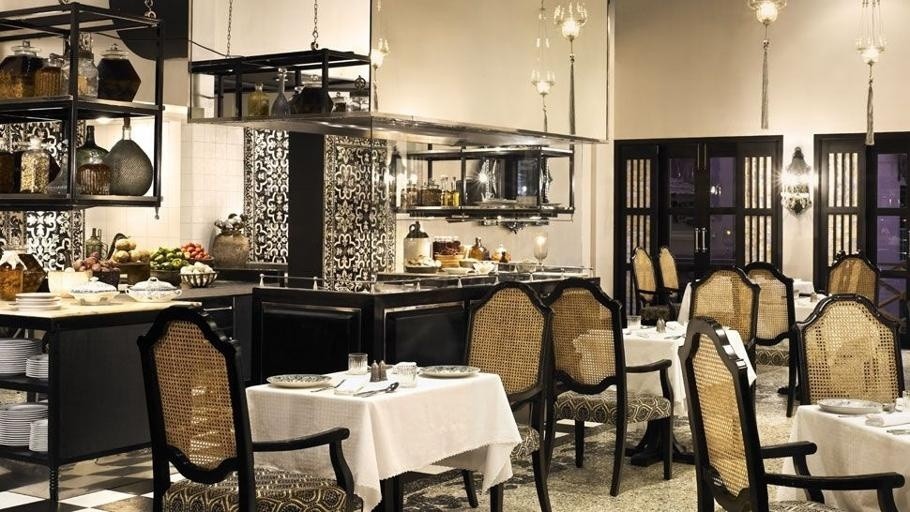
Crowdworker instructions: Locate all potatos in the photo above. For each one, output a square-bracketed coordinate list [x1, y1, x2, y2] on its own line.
[112, 238, 151, 263]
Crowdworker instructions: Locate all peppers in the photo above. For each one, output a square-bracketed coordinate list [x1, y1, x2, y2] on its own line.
[149, 246, 188, 271]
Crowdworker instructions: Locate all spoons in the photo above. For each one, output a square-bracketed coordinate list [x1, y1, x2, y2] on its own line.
[353, 382, 398, 398]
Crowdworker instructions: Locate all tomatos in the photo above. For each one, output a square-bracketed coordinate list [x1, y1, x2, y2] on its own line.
[181, 242, 211, 260]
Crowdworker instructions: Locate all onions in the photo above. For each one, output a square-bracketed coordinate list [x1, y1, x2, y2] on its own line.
[72, 251, 120, 273]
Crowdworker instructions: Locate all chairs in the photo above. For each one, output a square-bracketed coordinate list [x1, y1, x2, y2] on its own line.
[540, 276, 676, 498]
[135, 303, 365, 512]
[651, 243, 685, 321]
[689, 264, 762, 419]
[630, 245, 683, 328]
[678, 315, 910, 512]
[743, 260, 798, 418]
[790, 292, 904, 406]
[461, 280, 554, 512]
[825, 253, 881, 311]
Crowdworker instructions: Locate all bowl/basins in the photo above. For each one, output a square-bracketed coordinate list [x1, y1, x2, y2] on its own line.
[473, 263, 494, 274]
[458, 260, 476, 268]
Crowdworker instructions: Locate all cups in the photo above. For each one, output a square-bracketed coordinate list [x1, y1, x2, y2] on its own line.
[627, 316, 642, 330]
[397, 362, 417, 388]
[348, 353, 368, 374]
[901, 390, 909, 415]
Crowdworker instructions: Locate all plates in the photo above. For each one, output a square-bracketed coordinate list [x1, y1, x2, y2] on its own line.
[266, 374, 332, 388]
[817, 398, 883, 414]
[29, 418, 48, 452]
[0, 403, 48, 446]
[0, 338, 42, 378]
[442, 267, 472, 274]
[8, 293, 62, 311]
[435, 254, 465, 268]
[25, 354, 49, 383]
[419, 365, 481, 378]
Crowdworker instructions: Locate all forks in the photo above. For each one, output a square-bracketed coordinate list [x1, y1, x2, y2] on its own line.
[310, 379, 346, 392]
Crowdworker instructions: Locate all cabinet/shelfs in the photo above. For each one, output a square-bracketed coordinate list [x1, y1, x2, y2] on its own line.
[0, 0, 167, 220]
[187, 1, 611, 145]
[1, 299, 201, 507]
[395, 142, 576, 234]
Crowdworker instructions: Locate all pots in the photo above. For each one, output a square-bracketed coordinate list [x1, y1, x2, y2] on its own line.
[68, 277, 120, 305]
[124, 277, 182, 303]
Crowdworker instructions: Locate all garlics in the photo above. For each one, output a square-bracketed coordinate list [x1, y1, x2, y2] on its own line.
[180, 261, 217, 286]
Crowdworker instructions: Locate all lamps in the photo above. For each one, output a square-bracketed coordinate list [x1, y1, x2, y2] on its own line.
[371, 1, 389, 112]
[530, 1, 557, 133]
[533, 229, 549, 266]
[778, 146, 815, 218]
[856, 0, 891, 147]
[551, 1, 589, 135]
[746, 1, 789, 132]
